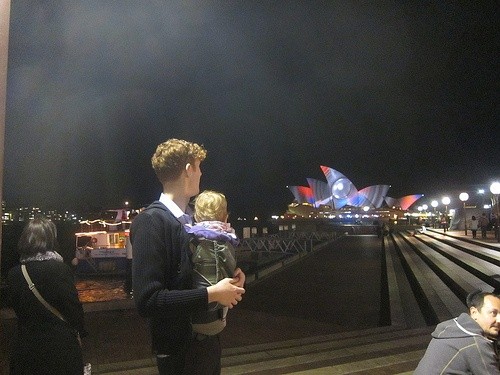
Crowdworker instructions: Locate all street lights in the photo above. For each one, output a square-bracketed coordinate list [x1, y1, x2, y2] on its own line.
[459, 192, 469, 236]
[490, 181, 500, 243]
[441, 196, 451, 231]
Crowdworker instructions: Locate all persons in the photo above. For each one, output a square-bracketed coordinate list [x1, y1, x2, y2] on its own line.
[130, 138, 246, 374]
[478, 212, 489, 239]
[414, 291, 500, 375]
[185, 191, 241, 335]
[470, 216, 479, 238]
[373, 214, 450, 238]
[492, 214, 500, 240]
[6, 214, 90, 375]
[129, 208, 138, 219]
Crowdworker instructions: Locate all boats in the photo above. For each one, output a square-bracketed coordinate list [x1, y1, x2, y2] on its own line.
[71, 231, 132, 273]
